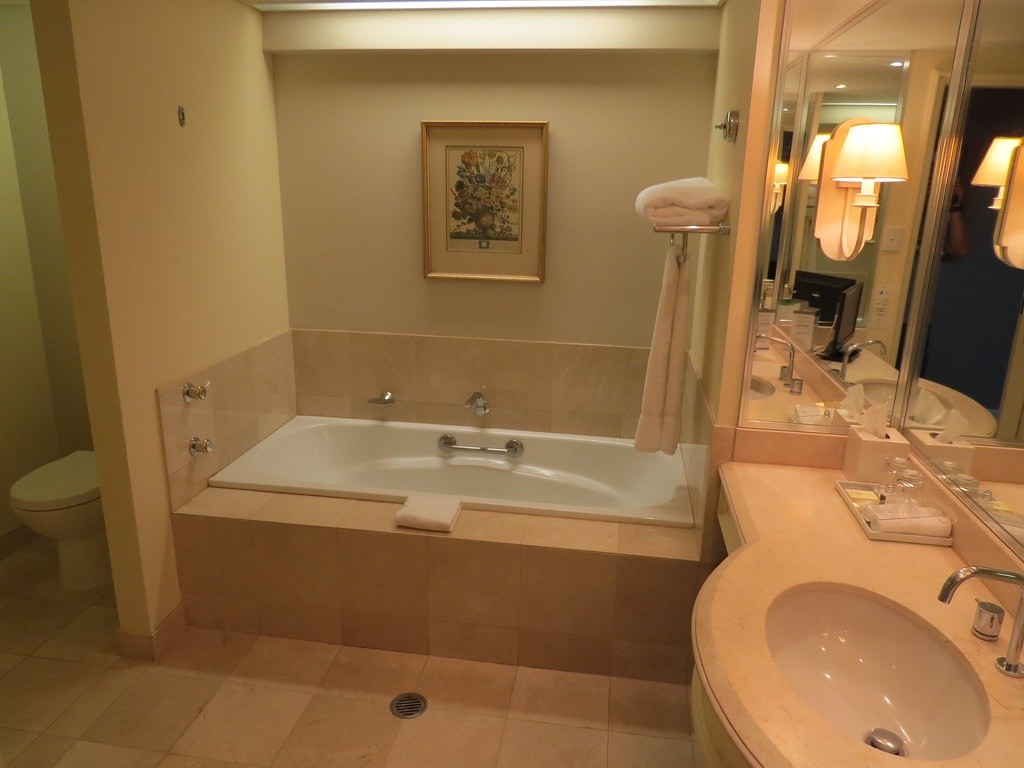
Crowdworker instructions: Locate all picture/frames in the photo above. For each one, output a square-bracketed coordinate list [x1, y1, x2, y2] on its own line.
[421, 118, 550, 284]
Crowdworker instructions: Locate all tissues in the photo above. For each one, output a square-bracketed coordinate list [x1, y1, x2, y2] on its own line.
[833, 383, 870, 426]
[910, 409, 975, 482]
[841, 403, 912, 486]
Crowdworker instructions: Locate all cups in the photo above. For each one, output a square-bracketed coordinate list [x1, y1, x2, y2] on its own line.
[891, 469, 925, 505]
[949, 471, 978, 500]
[936, 459, 962, 480]
[877, 457, 911, 498]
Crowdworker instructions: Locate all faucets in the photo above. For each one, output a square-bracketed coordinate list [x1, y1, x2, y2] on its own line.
[463, 391, 491, 418]
[840, 339, 886, 382]
[937, 565, 1024, 678]
[753, 332, 795, 388]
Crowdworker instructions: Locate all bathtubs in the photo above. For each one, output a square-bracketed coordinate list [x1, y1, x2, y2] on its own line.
[207, 414, 696, 529]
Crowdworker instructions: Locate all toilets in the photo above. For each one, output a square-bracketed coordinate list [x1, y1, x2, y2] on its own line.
[8, 449, 118, 595]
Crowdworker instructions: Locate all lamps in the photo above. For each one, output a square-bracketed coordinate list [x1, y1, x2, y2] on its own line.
[768, 159, 790, 215]
[814, 116, 912, 265]
[795, 131, 834, 184]
[970, 132, 1024, 272]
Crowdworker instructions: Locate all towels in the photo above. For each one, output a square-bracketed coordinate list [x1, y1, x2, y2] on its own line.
[632, 177, 730, 457]
[870, 516, 952, 537]
[393, 489, 465, 534]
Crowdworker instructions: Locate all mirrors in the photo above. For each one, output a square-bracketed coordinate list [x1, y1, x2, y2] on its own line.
[898, 1, 1023, 566]
[735, 1, 980, 439]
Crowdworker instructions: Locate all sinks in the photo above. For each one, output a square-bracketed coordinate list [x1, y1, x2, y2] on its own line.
[749, 373, 776, 401]
[764, 580, 992, 762]
[851, 378, 951, 430]
